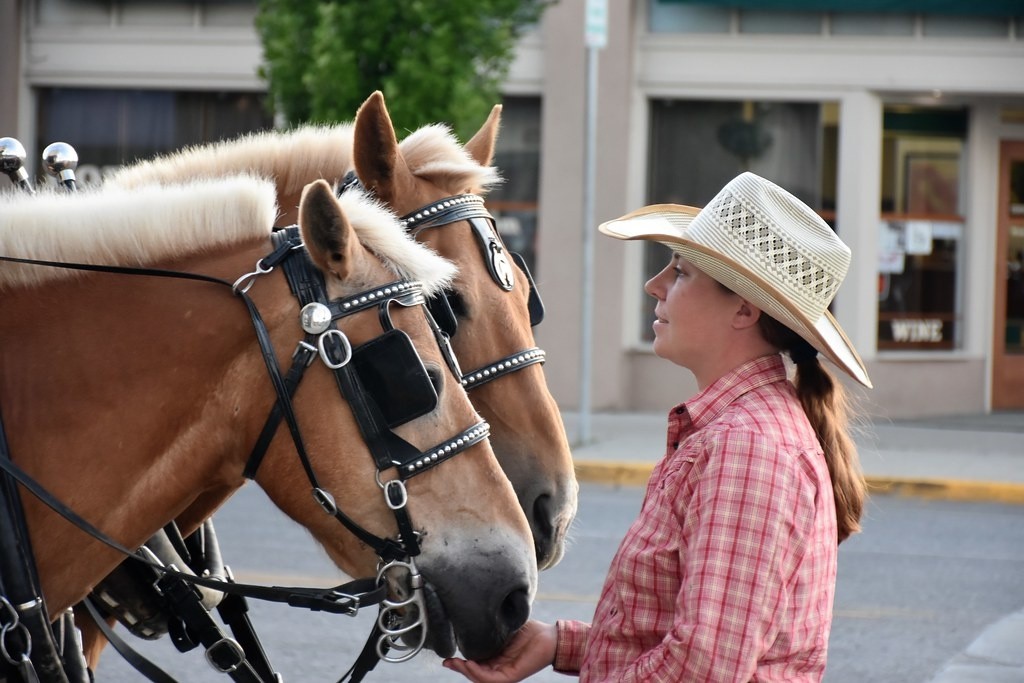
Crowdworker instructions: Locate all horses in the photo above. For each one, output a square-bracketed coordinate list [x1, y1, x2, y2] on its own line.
[0, 89, 581, 683]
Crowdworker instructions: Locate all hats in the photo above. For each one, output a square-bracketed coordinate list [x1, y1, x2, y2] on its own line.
[598, 171, 874, 390]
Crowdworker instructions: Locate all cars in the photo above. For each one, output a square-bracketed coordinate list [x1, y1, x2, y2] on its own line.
[876, 215, 961, 341]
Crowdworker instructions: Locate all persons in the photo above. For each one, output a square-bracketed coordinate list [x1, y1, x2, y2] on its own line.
[443, 171, 873, 683]
[876, 196, 905, 343]
